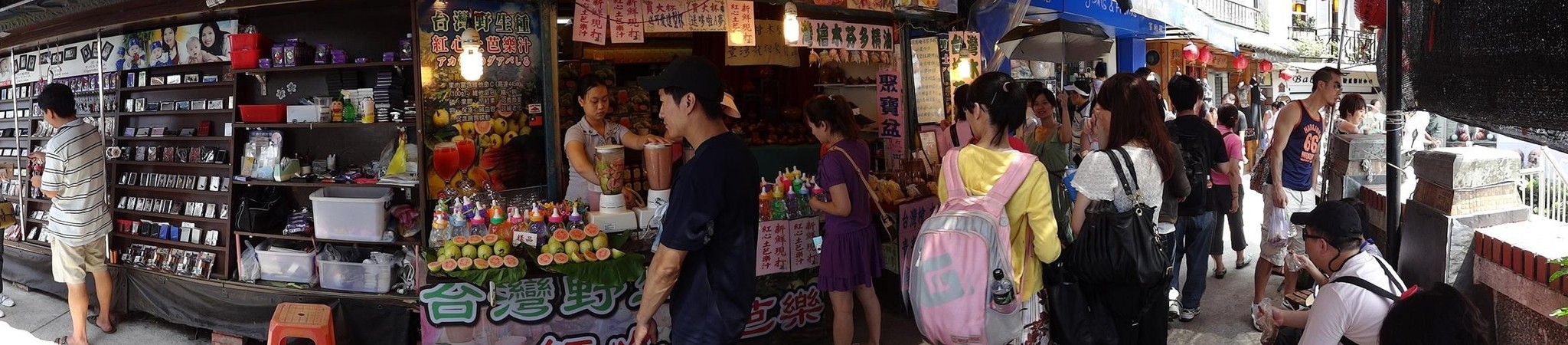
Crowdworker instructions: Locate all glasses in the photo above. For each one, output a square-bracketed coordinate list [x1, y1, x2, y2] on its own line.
[1325, 81, 1343, 92]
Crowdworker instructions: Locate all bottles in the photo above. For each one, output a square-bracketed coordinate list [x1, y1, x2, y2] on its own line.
[453, 196, 504, 219]
[468, 210, 488, 236]
[811, 183, 824, 216]
[452, 213, 468, 237]
[489, 209, 512, 242]
[428, 214, 451, 246]
[772, 186, 785, 219]
[759, 187, 771, 222]
[566, 207, 585, 231]
[32, 164, 43, 199]
[799, 184, 811, 217]
[342, 98, 355, 123]
[361, 97, 374, 125]
[433, 199, 448, 221]
[511, 208, 528, 236]
[760, 166, 819, 192]
[528, 209, 547, 249]
[787, 186, 799, 220]
[547, 208, 565, 236]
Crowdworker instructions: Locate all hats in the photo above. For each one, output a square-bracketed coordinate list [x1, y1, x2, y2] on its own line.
[636, 55, 726, 105]
[1063, 84, 1089, 96]
[1291, 200, 1362, 234]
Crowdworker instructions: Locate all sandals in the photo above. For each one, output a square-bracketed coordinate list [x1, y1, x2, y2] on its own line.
[1215, 267, 1227, 279]
[1236, 255, 1252, 268]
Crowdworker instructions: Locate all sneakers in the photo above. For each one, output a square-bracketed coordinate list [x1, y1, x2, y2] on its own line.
[1179, 306, 1201, 322]
[1166, 300, 1179, 318]
[0, 310, 4, 318]
[0, 293, 16, 308]
[1251, 297, 1265, 331]
[1281, 298, 1309, 311]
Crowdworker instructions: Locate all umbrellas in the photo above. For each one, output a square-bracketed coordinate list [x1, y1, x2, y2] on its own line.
[996, 18, 1114, 90]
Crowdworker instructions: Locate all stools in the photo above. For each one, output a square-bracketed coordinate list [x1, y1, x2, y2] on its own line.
[266, 302, 337, 345]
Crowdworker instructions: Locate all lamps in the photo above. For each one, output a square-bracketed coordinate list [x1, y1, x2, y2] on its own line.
[455, 1, 487, 82]
[779, 0, 802, 48]
[952, 0, 976, 82]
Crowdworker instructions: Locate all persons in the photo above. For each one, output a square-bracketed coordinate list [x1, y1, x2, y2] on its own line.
[93, 44, 98, 56]
[186, 36, 215, 64]
[1093, 62, 1108, 94]
[102, 47, 110, 58]
[1402, 110, 1430, 150]
[1366, 99, 1386, 133]
[1261, 101, 1278, 152]
[54, 55, 59, 62]
[29, 58, 34, 69]
[1161, 73, 1230, 322]
[0, 58, 11, 82]
[1291, 197, 1383, 288]
[1221, 93, 1248, 181]
[1251, 66, 1346, 332]
[160, 26, 188, 66]
[941, 84, 975, 157]
[1255, 201, 1409, 345]
[801, 94, 896, 344]
[29, 83, 117, 345]
[148, 40, 172, 66]
[1328, 93, 1368, 165]
[69, 50, 74, 57]
[907, 71, 1062, 345]
[116, 47, 126, 71]
[1016, 82, 1046, 146]
[1200, 78, 1213, 109]
[1234, 81, 1251, 136]
[1206, 105, 1218, 129]
[1141, 81, 1168, 124]
[0, 171, 16, 319]
[564, 74, 674, 205]
[123, 38, 146, 69]
[1072, 73, 1173, 345]
[199, 22, 231, 63]
[1210, 103, 1251, 278]
[1065, 78, 1091, 160]
[83, 48, 90, 60]
[1249, 77, 1261, 137]
[633, 55, 757, 345]
[1378, 282, 1489, 345]
[1028, 86, 1073, 246]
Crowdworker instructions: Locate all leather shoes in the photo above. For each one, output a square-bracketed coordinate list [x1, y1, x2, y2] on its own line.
[1272, 266, 1285, 276]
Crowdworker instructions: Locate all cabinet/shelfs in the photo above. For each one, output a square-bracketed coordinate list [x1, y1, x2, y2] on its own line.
[0, 61, 419, 294]
[810, 46, 893, 147]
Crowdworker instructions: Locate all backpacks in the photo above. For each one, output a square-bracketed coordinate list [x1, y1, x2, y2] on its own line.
[1167, 120, 1212, 210]
[1330, 256, 1424, 345]
[907, 145, 1040, 345]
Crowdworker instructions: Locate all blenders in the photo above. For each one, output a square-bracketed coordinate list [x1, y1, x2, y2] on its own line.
[584, 144, 637, 231]
[631, 142, 674, 230]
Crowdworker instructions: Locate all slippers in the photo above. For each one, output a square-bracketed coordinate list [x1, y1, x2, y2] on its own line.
[53, 335, 69, 345]
[87, 314, 117, 334]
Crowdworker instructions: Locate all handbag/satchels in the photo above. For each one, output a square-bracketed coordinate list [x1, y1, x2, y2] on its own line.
[1067, 146, 1174, 289]
